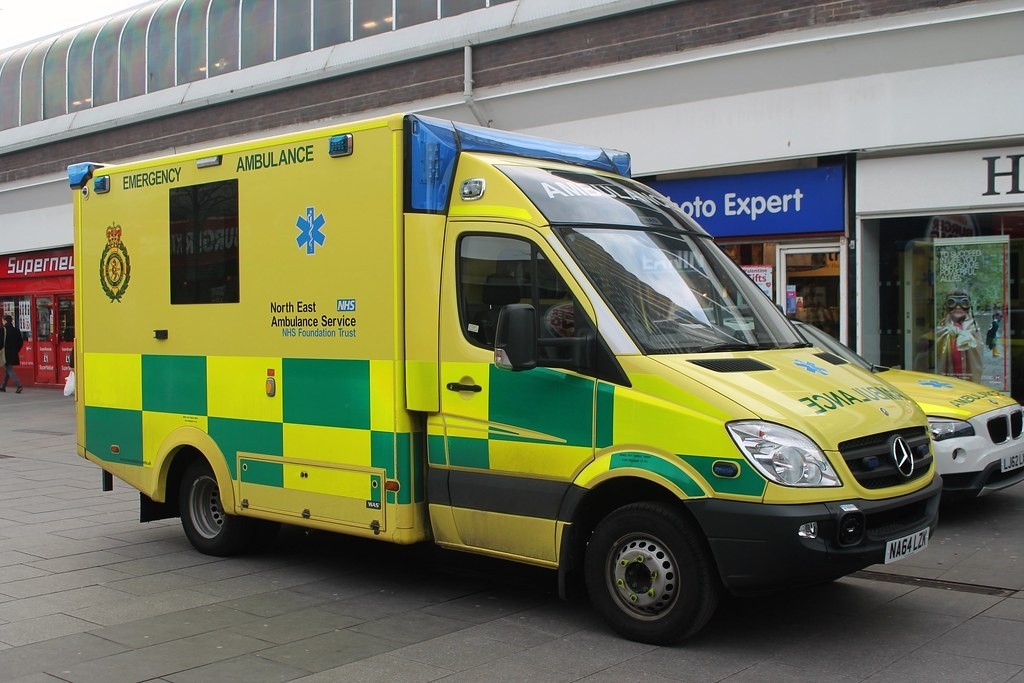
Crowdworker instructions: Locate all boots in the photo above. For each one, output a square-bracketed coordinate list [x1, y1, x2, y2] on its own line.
[993, 346, 1001, 357]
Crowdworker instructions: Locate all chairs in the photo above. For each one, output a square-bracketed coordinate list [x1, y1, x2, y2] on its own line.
[469, 273, 522, 348]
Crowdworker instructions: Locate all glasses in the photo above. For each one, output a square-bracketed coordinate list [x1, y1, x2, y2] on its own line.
[946, 297, 971, 311]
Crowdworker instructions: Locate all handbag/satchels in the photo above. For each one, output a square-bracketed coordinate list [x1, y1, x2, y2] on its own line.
[64, 370, 75, 396]
[0, 348, 6, 367]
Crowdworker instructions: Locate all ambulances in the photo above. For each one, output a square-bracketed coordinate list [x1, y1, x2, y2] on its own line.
[787, 319, 1022, 502]
[67, 113, 944, 644]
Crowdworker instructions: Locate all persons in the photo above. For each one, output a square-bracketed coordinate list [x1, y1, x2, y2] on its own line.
[986, 315, 1000, 357]
[0, 315, 23, 393]
[467, 273, 521, 343]
[936, 288, 984, 385]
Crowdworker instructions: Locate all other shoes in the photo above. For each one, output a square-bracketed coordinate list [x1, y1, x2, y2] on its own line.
[0, 386, 6, 392]
[15, 386, 23, 393]
